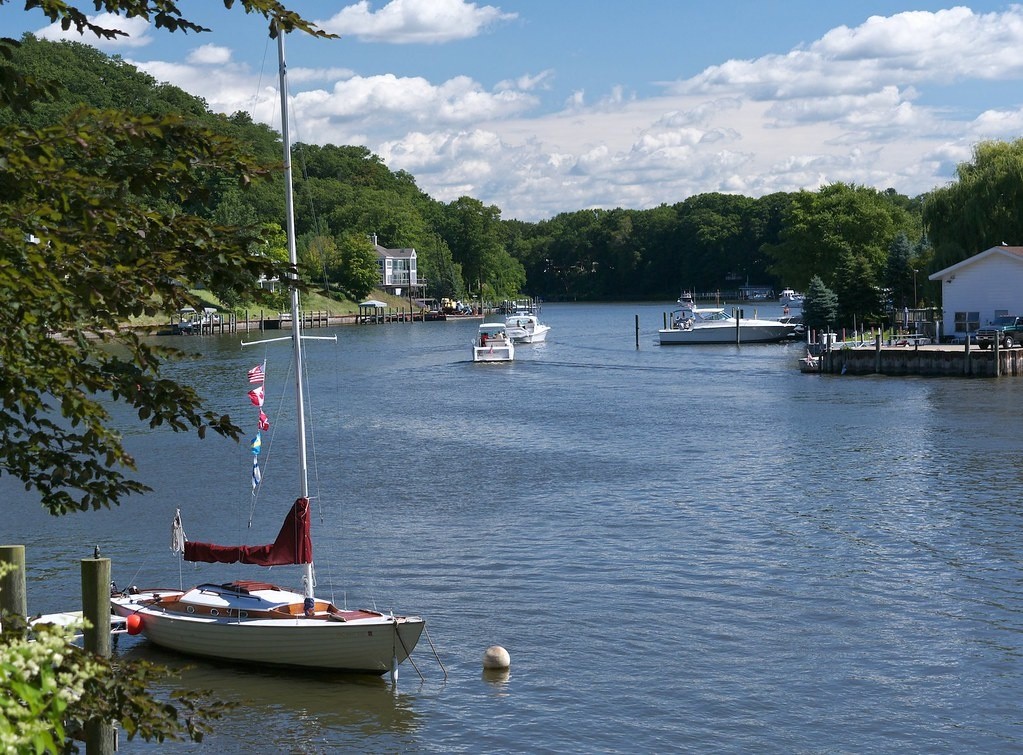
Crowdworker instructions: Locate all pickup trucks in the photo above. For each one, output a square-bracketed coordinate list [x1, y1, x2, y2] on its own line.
[974, 315, 1023, 349]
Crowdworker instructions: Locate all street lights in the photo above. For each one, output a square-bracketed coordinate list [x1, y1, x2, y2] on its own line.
[914, 270, 919, 308]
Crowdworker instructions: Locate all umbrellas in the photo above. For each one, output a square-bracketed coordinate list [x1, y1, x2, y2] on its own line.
[172, 504, 189, 593]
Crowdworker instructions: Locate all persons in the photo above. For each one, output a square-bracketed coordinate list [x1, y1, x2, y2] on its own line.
[496, 330, 503, 340]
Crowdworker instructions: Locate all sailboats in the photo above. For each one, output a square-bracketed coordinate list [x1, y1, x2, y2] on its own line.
[108, 20, 426, 680]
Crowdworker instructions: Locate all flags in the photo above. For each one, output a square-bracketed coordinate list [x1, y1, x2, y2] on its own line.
[247, 384, 265, 407]
[245, 361, 268, 384]
[249, 430, 261, 456]
[257, 409, 269, 431]
[251, 456, 262, 495]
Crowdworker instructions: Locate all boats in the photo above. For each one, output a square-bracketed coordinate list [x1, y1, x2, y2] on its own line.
[470, 322, 514, 362]
[658, 307, 799, 344]
[676, 287, 697, 311]
[178, 306, 220, 336]
[504, 310, 550, 342]
[22, 608, 148, 663]
[512, 297, 535, 314]
[779, 287, 804, 309]
